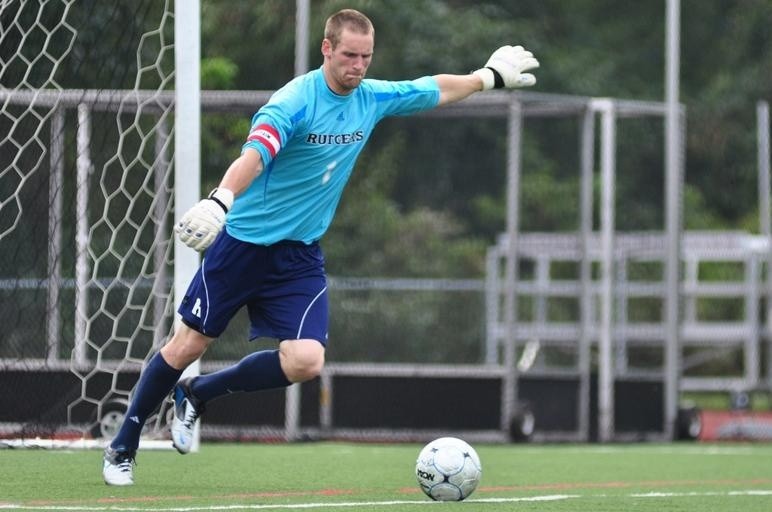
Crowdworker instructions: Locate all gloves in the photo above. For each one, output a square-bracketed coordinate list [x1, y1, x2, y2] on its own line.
[474, 42, 545, 93]
[172, 185, 241, 252]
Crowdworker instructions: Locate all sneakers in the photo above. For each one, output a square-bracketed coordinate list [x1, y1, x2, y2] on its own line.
[101, 433, 139, 490]
[169, 373, 205, 458]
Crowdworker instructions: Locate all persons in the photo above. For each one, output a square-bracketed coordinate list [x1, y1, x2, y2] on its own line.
[101, 8, 540, 484]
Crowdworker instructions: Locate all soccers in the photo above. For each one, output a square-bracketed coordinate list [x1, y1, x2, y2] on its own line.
[417, 437, 481, 502]
